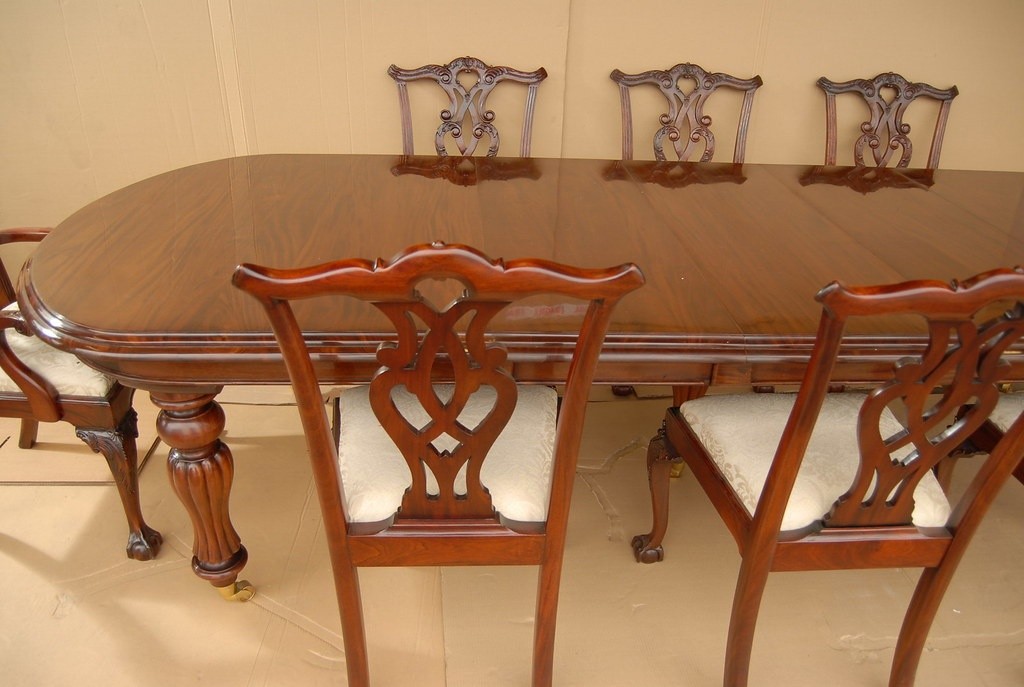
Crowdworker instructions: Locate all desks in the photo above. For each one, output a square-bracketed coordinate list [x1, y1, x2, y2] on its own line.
[17, 154, 1024, 588]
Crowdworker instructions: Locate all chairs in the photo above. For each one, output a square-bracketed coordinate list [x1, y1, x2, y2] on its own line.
[610, 60, 776, 407]
[0, 230, 162, 559]
[817, 70, 961, 392]
[631, 267, 1024, 687]
[230, 248, 644, 687]
[388, 56, 549, 157]
[933, 381, 1024, 496]
